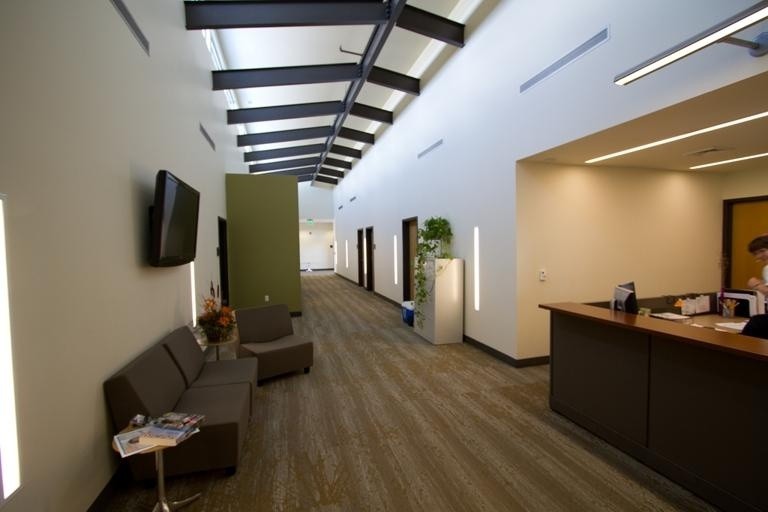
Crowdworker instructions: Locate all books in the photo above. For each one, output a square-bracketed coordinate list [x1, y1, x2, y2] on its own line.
[714, 321, 747, 331]
[114, 423, 202, 458]
[716, 286, 765, 319]
[137, 410, 206, 446]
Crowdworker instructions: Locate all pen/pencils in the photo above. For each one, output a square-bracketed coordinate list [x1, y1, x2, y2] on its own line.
[720, 298, 740, 318]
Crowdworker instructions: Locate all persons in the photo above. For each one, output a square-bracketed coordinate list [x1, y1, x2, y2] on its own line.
[747, 232, 768, 313]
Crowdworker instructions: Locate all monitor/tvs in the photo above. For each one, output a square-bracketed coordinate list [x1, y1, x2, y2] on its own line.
[617, 281, 636, 292]
[148, 170, 201, 267]
[611, 286, 638, 314]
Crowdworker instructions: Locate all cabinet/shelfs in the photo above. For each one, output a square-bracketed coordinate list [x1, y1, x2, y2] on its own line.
[411, 256, 464, 347]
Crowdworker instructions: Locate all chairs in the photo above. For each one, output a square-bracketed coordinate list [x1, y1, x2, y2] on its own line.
[109, 299, 317, 480]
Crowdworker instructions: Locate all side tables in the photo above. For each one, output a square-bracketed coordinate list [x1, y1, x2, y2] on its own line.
[109, 412, 203, 512]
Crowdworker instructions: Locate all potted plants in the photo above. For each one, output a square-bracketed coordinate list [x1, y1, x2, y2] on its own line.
[412, 215, 456, 328]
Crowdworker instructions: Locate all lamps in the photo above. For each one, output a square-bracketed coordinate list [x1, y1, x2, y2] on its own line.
[612, 0, 766, 89]
